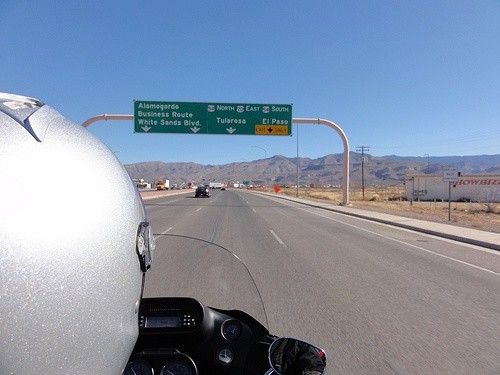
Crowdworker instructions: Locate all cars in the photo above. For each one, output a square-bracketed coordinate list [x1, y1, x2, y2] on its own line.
[194, 186, 210, 198]
[220, 187, 225, 191]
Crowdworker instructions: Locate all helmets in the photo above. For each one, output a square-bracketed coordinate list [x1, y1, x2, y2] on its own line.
[0, 92, 153, 375]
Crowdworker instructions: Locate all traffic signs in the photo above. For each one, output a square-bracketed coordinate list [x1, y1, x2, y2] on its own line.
[133, 101, 292, 136]
